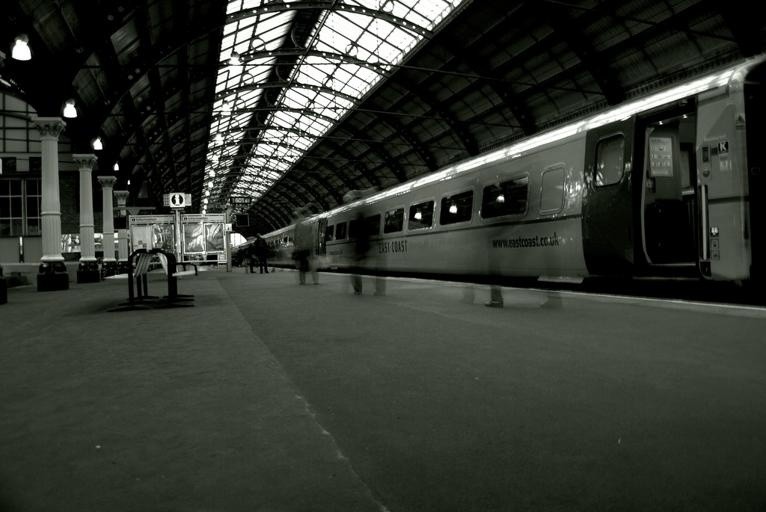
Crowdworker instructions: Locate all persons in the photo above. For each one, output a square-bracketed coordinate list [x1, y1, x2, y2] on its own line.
[232, 232, 270, 274]
[293, 221, 321, 286]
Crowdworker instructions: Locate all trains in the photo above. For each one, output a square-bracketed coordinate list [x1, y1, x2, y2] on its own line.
[229, 53, 764, 307]
